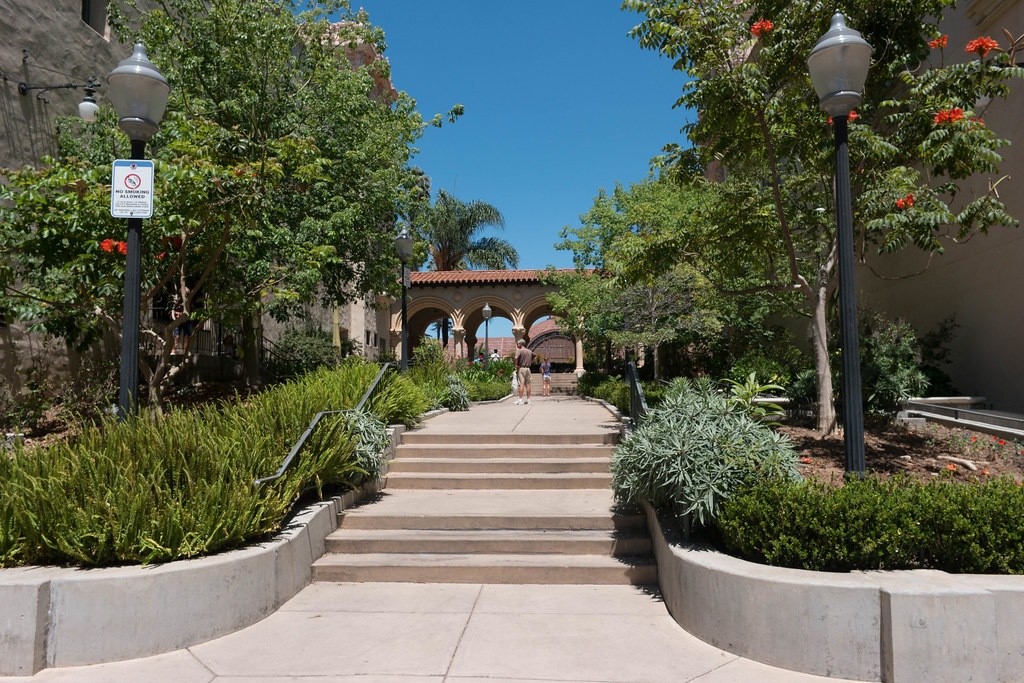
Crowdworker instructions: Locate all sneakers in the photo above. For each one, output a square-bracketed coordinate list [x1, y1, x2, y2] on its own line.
[512, 398, 521, 406]
[524, 399, 529, 404]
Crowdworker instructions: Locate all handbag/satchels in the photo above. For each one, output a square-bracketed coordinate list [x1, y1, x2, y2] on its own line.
[511, 369, 517, 390]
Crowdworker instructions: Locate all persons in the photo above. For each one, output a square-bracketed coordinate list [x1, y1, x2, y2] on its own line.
[465, 348, 487, 367]
[490, 349, 501, 364]
[539, 356, 551, 396]
[513, 338, 538, 404]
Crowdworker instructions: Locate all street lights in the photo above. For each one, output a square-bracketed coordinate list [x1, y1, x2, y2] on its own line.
[804, 9, 873, 482]
[105, 41, 172, 423]
[482, 302, 492, 370]
[394, 226, 414, 370]
